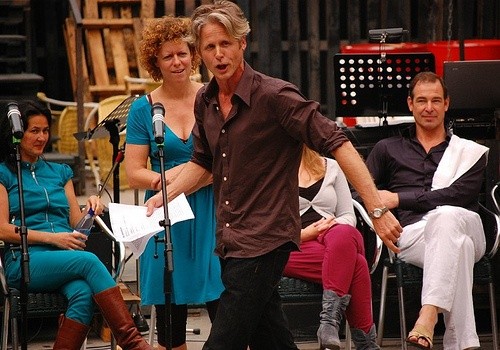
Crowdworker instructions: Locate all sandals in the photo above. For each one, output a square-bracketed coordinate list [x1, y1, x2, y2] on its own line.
[406, 315, 439, 350]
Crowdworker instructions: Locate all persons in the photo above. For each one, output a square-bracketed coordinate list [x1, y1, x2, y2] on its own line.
[281, 143, 382, 350]
[142, 0, 402, 350]
[0, 99, 154, 350]
[124, 13, 226, 350]
[350, 72, 500, 350]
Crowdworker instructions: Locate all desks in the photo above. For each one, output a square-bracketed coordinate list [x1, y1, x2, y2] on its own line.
[42, 152, 87, 198]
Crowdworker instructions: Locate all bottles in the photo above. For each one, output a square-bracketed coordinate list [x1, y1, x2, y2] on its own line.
[72, 208, 94, 250]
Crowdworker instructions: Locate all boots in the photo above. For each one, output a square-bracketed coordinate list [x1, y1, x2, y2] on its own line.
[318, 289, 351, 350]
[350, 323, 380, 350]
[53, 313, 92, 350]
[93, 286, 154, 350]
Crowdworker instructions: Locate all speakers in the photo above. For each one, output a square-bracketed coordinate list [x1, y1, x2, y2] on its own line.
[442, 59, 500, 265]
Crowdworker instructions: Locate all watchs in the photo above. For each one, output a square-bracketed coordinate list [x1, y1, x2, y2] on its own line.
[368, 207, 388, 219]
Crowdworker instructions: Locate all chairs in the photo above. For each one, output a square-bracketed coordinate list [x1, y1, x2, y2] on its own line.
[124, 74, 203, 95]
[83, 95, 152, 199]
[378, 199, 500, 350]
[56, 106, 98, 157]
[277, 199, 383, 350]
[0, 213, 121, 350]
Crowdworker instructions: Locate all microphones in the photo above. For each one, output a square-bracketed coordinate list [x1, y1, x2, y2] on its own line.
[151, 101, 165, 145]
[6, 100, 25, 139]
